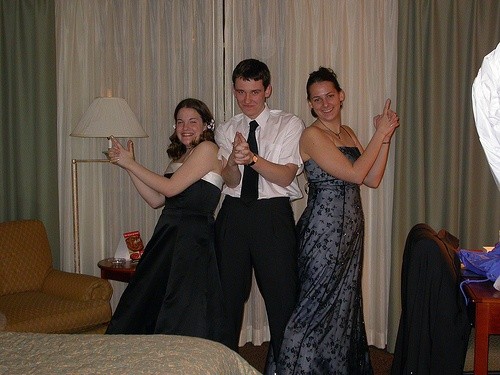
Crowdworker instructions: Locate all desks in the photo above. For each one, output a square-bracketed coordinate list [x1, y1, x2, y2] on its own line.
[454, 249, 500, 375]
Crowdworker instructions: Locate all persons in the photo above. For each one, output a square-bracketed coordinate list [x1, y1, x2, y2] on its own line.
[211, 59, 307, 363]
[264, 67, 401, 375]
[105, 98, 225, 340]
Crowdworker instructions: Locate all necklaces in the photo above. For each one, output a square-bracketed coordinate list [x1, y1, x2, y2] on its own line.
[318, 118, 341, 140]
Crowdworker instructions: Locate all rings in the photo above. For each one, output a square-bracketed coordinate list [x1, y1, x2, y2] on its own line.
[391, 124, 392, 127]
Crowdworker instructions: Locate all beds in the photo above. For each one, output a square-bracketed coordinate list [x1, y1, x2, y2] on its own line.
[0, 332, 263, 375]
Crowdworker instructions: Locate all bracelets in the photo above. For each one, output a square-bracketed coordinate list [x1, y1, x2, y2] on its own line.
[382, 142, 390, 144]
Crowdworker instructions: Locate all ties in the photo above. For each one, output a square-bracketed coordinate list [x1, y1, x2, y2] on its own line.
[239, 120, 260, 205]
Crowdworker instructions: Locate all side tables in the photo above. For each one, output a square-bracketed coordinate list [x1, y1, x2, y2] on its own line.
[98, 257, 136, 326]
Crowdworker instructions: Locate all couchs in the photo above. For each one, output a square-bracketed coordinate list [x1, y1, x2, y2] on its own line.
[0, 219, 111, 334]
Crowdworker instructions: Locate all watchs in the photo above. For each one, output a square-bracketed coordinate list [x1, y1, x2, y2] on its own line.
[248, 153, 258, 166]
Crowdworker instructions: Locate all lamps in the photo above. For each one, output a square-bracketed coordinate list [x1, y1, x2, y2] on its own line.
[68, 97, 149, 273]
